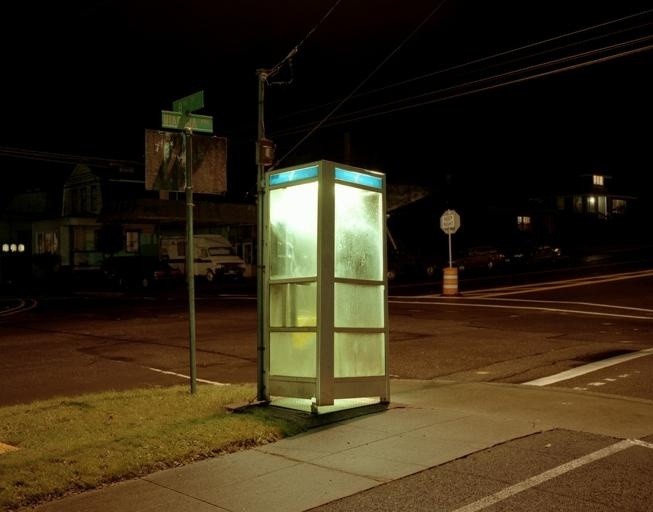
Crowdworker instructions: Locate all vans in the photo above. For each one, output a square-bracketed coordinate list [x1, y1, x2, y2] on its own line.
[160, 233, 246, 281]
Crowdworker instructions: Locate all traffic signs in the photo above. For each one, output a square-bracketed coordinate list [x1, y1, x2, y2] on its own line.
[172, 89, 204, 114]
[161, 110, 212, 133]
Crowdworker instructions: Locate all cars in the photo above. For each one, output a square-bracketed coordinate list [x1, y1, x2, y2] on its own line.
[388, 243, 562, 272]
[96, 256, 166, 288]
